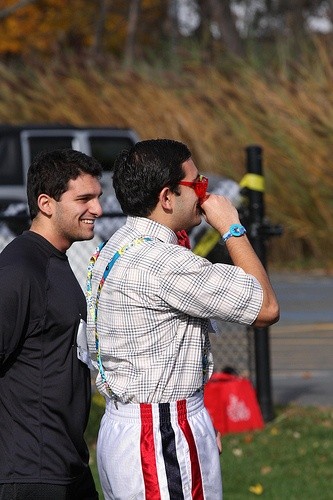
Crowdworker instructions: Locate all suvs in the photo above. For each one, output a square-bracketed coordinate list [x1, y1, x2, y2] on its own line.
[0, 124, 144, 241]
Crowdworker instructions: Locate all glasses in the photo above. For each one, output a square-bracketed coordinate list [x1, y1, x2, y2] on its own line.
[158, 174, 210, 198]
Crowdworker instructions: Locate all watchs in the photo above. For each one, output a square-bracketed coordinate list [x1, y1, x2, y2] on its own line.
[222, 223, 246, 242]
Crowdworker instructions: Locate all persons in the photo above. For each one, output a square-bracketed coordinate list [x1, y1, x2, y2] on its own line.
[86, 136, 281, 500]
[0, 147, 103, 500]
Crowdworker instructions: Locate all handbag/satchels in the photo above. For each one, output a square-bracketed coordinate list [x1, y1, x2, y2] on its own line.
[202, 373, 265, 434]
